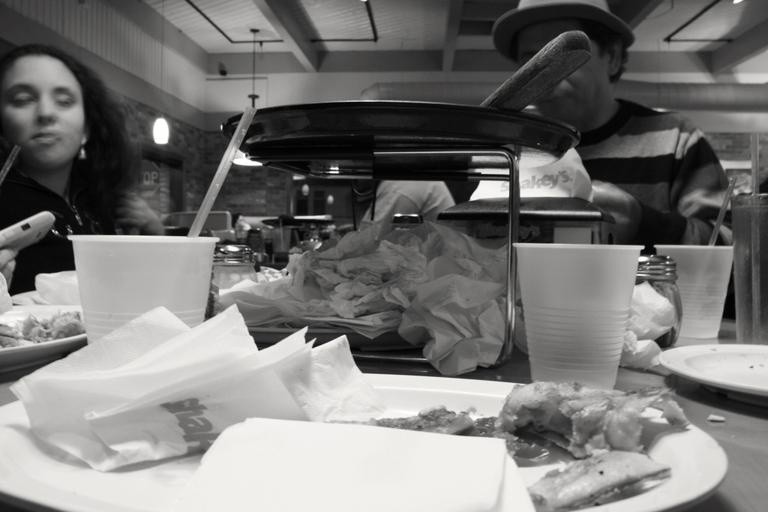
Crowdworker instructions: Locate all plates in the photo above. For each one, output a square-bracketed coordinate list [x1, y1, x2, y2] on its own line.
[0, 305, 88, 372]
[0, 368, 731, 512]
[246, 325, 396, 351]
[221, 99, 584, 169]
[658, 343, 768, 397]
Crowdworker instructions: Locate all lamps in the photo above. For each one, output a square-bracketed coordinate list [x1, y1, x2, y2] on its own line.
[230, 28, 264, 166]
[153, 0, 169, 145]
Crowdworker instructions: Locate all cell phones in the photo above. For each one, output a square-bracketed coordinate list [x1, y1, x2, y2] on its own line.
[0, 211, 57, 251]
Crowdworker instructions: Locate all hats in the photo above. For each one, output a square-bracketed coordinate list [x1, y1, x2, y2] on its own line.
[492, 0, 635, 62]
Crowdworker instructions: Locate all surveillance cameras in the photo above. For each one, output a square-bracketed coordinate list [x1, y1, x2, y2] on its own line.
[217, 63, 227, 77]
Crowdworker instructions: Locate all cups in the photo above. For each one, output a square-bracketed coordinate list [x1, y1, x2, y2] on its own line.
[656, 244, 734, 340]
[512, 240, 645, 392]
[732, 193, 768, 346]
[68, 232, 220, 351]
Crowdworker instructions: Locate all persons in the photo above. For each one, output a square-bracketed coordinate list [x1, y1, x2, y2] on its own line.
[0, 43, 163, 298]
[491, 0, 733, 246]
[360, 181, 457, 232]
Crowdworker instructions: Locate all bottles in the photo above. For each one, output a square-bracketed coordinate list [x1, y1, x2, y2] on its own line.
[634, 255, 683, 348]
[212, 243, 258, 318]
